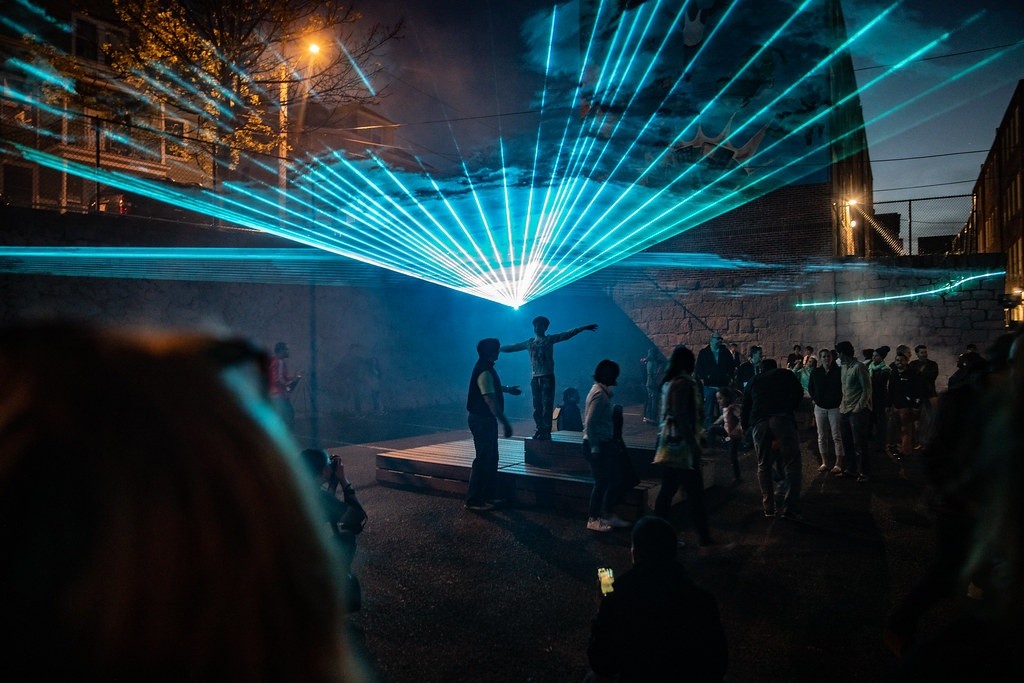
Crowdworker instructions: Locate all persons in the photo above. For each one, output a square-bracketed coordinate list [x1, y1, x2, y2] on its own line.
[500, 318, 598, 439]
[588, 516, 728, 683]
[646, 348, 736, 557]
[882, 332, 1023, 668]
[465, 339, 520, 510]
[643, 332, 988, 483]
[558, 388, 585, 432]
[580, 360, 630, 534]
[0, 271, 368, 683]
[741, 358, 805, 517]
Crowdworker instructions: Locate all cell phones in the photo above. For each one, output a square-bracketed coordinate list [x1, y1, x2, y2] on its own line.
[597, 566, 614, 594]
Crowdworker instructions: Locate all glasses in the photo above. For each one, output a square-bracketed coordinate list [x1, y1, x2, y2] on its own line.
[713, 336, 723, 340]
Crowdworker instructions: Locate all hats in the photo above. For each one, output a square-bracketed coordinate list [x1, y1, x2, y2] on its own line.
[875, 345, 890, 361]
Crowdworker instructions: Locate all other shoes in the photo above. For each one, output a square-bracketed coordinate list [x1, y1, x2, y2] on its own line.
[602, 514, 632, 528]
[915, 446, 929, 454]
[893, 451, 912, 462]
[587, 517, 612, 532]
[835, 467, 859, 479]
[730, 476, 743, 489]
[831, 466, 843, 473]
[784, 510, 807, 523]
[488, 496, 505, 507]
[858, 473, 868, 484]
[817, 464, 829, 472]
[643, 416, 658, 427]
[533, 430, 552, 441]
[463, 501, 494, 511]
